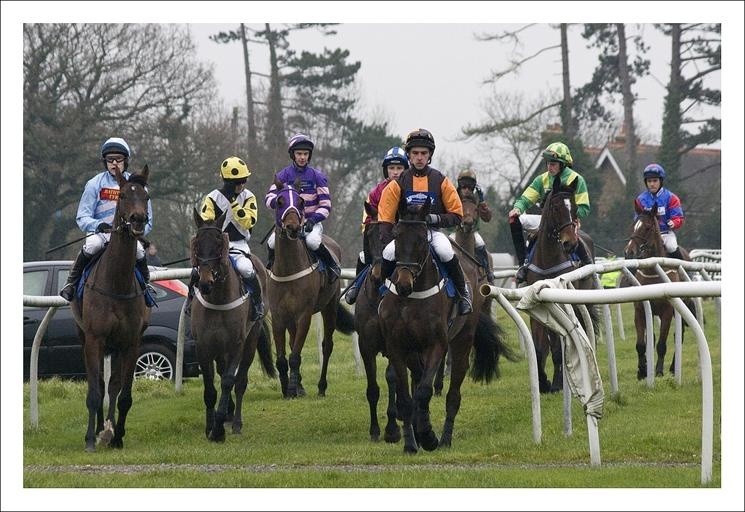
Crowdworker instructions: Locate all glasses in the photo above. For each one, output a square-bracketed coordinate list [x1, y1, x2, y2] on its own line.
[459, 183, 474, 189]
[104, 156, 126, 162]
[232, 178, 247, 185]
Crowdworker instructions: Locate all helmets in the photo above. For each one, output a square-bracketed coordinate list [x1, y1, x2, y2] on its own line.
[102, 137, 130, 156]
[457, 167, 477, 182]
[541, 142, 573, 167]
[288, 133, 313, 161]
[383, 146, 409, 178]
[404, 128, 435, 158]
[220, 156, 251, 179]
[644, 164, 664, 183]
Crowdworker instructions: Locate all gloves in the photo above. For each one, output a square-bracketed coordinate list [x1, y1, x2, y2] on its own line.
[426, 214, 440, 226]
[140, 236, 149, 246]
[223, 182, 236, 203]
[303, 219, 315, 232]
[97, 222, 111, 233]
[475, 185, 484, 202]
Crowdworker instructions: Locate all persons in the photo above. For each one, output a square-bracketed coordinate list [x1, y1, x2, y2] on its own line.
[507, 141, 591, 282]
[264, 134, 341, 284]
[634, 163, 685, 260]
[355, 145, 411, 277]
[449, 170, 495, 282]
[184, 157, 265, 321]
[60, 135, 157, 308]
[345, 130, 472, 316]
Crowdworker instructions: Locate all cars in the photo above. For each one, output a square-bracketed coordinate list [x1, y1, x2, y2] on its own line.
[23, 261, 200, 385]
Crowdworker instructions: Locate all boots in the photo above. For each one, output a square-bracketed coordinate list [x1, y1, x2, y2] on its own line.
[576, 240, 593, 281]
[60, 249, 93, 301]
[510, 216, 526, 281]
[476, 245, 494, 282]
[183, 268, 199, 316]
[669, 247, 683, 260]
[620, 251, 637, 287]
[313, 242, 341, 284]
[243, 274, 265, 321]
[442, 255, 472, 315]
[266, 245, 274, 270]
[135, 254, 157, 307]
[345, 257, 366, 305]
[380, 258, 396, 283]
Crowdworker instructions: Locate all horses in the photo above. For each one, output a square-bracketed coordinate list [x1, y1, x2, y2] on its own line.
[267, 174, 355, 403]
[446, 192, 495, 375]
[70, 163, 153, 455]
[523, 174, 603, 394]
[617, 200, 706, 382]
[187, 206, 278, 439]
[353, 199, 415, 443]
[380, 190, 521, 452]
